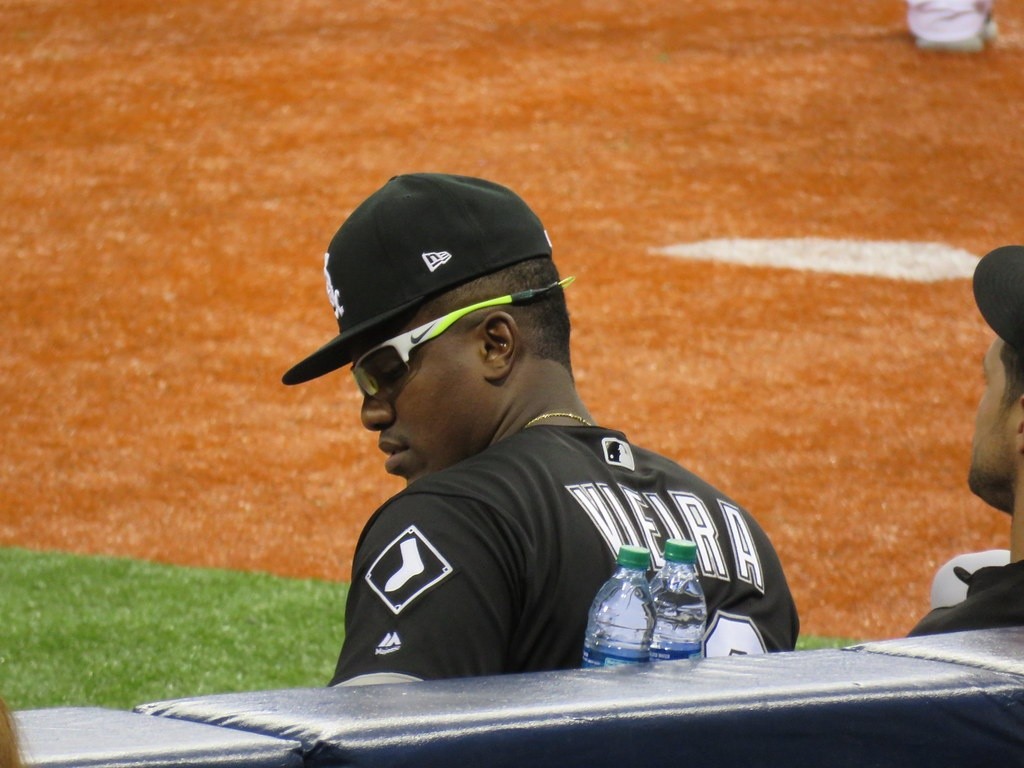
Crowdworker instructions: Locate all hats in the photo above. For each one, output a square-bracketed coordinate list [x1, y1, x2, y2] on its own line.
[973, 244, 1023, 352]
[282, 173, 553, 387]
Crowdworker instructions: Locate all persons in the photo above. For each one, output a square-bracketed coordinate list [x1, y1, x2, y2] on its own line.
[905, 0, 997, 54]
[902, 244, 1024, 637]
[279, 170, 803, 685]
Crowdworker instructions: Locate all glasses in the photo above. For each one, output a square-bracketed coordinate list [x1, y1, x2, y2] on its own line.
[350, 278, 575, 395]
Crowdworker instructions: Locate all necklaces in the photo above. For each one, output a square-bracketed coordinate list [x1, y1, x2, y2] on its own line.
[524, 413, 593, 428]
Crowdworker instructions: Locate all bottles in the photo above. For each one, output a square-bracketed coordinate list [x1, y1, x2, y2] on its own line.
[649, 540, 707, 662]
[582, 547, 655, 668]
